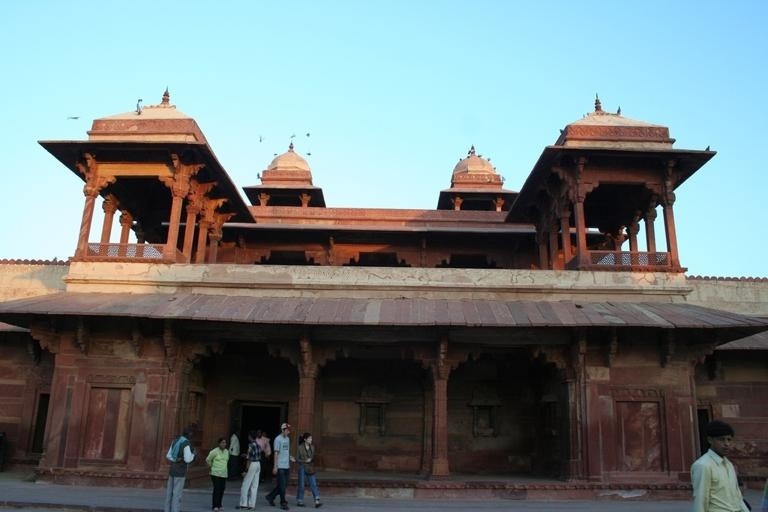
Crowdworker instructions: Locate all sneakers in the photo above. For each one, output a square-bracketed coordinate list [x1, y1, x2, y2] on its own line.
[265, 495, 322, 510]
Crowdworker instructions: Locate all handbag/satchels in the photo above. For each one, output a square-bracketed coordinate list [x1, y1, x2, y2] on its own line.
[304, 463, 316, 475]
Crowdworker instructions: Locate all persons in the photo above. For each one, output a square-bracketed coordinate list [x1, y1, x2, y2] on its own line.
[688, 419, 753, 510]
[265, 423, 294, 510]
[163, 428, 200, 512]
[206, 437, 233, 510]
[229, 426, 274, 512]
[290, 431, 325, 509]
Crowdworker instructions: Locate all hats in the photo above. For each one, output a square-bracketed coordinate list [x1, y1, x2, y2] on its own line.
[281, 423, 291, 429]
